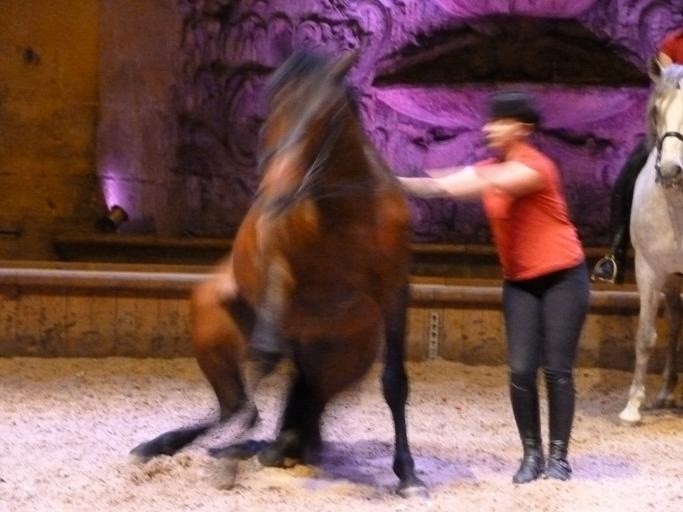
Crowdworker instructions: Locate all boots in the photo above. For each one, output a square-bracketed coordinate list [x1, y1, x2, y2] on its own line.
[547, 439, 572, 481]
[513, 438, 544, 483]
[593, 224, 630, 285]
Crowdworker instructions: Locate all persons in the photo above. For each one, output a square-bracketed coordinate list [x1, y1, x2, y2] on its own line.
[395, 91, 590, 483]
[592, 27, 683, 284]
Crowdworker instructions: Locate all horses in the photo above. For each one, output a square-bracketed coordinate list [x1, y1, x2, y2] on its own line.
[616, 52, 683, 427]
[123, 33, 430, 500]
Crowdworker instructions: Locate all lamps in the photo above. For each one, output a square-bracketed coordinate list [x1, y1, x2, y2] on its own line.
[103, 204, 128, 233]
